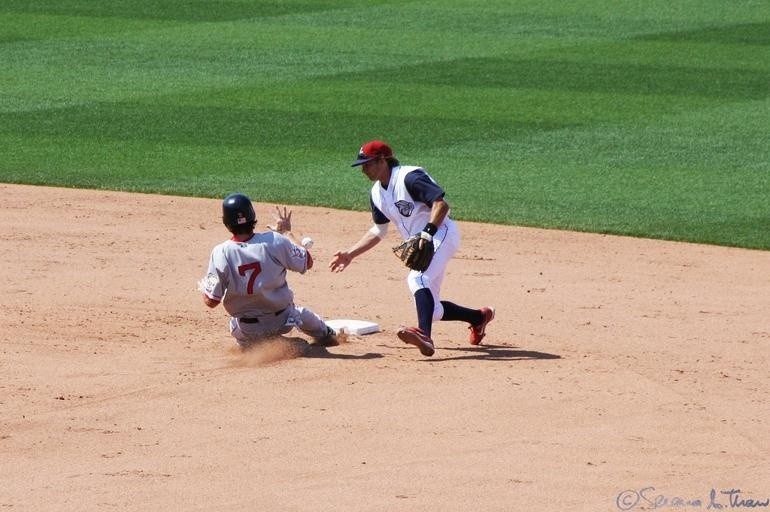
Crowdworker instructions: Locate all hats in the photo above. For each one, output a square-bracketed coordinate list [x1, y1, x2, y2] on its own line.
[351, 141, 393, 166]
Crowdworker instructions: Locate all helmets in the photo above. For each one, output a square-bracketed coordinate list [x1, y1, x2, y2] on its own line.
[223, 193, 255, 225]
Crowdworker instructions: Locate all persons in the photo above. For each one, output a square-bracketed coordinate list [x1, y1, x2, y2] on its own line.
[328, 141, 495, 355]
[198, 195, 343, 348]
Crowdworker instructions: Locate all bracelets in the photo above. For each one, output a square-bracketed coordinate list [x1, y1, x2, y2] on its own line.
[423, 223, 438, 236]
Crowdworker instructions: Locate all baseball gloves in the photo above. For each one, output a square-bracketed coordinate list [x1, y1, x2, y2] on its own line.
[392, 232, 434, 272]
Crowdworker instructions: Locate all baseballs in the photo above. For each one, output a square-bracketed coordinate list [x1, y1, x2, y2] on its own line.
[302, 238, 312, 248]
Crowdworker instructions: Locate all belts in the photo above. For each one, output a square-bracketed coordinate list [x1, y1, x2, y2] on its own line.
[240, 309, 285, 323]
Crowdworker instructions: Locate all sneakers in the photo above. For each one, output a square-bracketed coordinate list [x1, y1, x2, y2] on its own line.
[327, 326, 336, 336]
[468, 307, 495, 345]
[397, 327, 434, 356]
